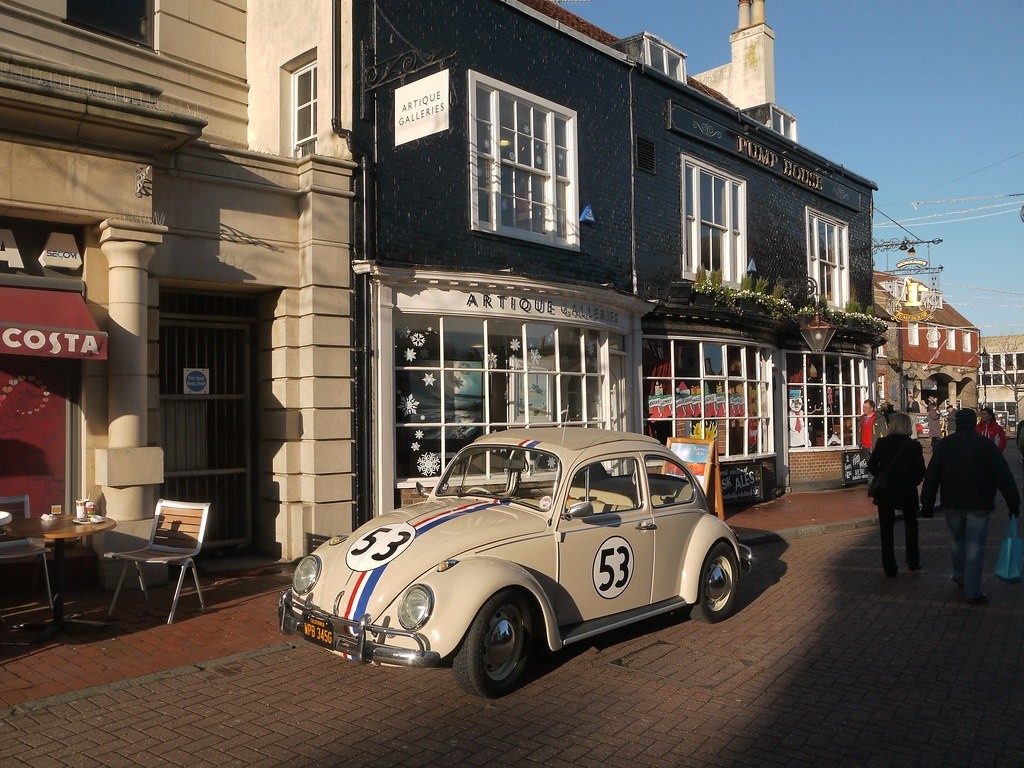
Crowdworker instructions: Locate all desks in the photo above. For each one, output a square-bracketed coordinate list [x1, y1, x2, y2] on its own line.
[4, 513, 116, 646]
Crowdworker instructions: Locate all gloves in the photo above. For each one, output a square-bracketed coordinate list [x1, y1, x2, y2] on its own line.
[920, 506, 934, 518]
[1008, 503, 1020, 520]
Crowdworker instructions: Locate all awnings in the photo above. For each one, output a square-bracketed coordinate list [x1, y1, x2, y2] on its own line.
[0, 272, 108, 359]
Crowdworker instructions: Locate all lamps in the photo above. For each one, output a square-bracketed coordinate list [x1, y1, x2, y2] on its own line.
[775, 276, 837, 354]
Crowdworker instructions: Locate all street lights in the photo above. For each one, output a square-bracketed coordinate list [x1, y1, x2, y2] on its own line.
[978, 347, 990, 409]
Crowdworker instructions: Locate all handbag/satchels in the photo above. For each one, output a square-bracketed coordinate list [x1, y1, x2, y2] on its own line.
[993, 513, 1024, 583]
[867, 476, 889, 509]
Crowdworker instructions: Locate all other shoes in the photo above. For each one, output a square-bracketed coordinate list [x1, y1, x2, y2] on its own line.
[908, 564, 923, 571]
[885, 565, 898, 578]
[952, 575, 964, 589]
[967, 592, 989, 605]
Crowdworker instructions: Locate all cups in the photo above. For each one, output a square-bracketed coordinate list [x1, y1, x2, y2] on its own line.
[86, 507, 96, 517]
[76, 499, 90, 518]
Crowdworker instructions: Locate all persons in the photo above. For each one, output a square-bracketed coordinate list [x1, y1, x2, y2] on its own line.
[944, 405, 958, 436]
[928, 405, 942, 450]
[921, 408, 1020, 605]
[857, 400, 888, 497]
[907, 396, 960, 413]
[868, 413, 926, 578]
[975, 408, 1007, 452]
[1016, 420, 1024, 454]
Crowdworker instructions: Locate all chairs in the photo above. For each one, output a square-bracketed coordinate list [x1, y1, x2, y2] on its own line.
[0, 495, 54, 622]
[103, 498, 212, 624]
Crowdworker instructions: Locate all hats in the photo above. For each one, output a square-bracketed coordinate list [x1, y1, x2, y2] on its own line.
[955, 408, 977, 425]
[946, 405, 953, 409]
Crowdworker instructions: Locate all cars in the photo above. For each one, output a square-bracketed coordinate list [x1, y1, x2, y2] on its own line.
[280, 427, 742, 700]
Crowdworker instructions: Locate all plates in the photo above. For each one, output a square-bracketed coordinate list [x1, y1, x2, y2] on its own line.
[73, 515, 92, 525]
